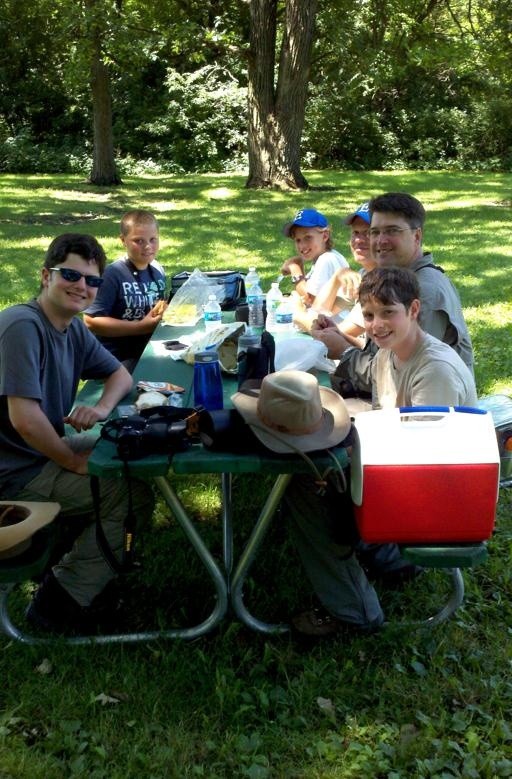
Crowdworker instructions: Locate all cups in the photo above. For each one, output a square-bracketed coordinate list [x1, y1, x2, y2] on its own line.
[237, 336, 261, 361]
[194, 352, 223, 411]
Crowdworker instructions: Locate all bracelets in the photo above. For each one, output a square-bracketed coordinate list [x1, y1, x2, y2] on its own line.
[290, 272, 305, 286]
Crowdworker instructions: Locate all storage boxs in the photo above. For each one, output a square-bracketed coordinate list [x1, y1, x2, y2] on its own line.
[352, 405, 501, 545]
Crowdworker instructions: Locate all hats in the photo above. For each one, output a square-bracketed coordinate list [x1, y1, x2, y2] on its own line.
[343, 202, 371, 225]
[0, 501, 62, 561]
[283, 208, 328, 237]
[230, 366, 352, 454]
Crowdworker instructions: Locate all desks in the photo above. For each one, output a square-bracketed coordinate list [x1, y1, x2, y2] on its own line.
[89, 287, 349, 649]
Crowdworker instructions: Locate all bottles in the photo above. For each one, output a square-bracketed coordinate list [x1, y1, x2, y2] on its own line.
[204, 265, 294, 336]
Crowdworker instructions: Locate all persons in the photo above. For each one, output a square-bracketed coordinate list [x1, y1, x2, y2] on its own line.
[279, 212, 353, 310]
[309, 191, 478, 408]
[80, 208, 167, 376]
[293, 201, 382, 349]
[292, 268, 479, 628]
[0, 232, 138, 629]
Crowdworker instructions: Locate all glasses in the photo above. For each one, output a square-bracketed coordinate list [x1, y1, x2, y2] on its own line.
[366, 227, 417, 240]
[47, 267, 105, 289]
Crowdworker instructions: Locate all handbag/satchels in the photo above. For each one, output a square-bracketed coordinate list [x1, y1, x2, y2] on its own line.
[100, 405, 209, 453]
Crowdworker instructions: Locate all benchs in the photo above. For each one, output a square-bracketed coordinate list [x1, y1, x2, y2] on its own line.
[350, 540, 489, 635]
[0, 378, 110, 646]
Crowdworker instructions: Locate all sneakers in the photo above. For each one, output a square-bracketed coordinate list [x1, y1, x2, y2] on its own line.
[290, 606, 380, 636]
[382, 565, 423, 593]
